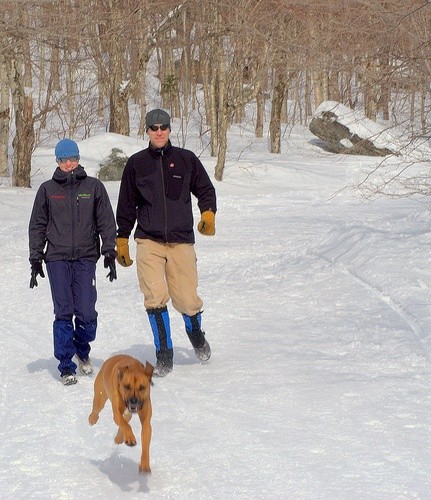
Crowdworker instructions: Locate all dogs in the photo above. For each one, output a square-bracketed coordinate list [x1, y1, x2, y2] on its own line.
[89, 354, 155, 473]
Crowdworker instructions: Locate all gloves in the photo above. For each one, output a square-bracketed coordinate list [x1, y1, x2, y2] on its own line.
[198, 211, 216, 235]
[115, 238, 132, 267]
[105, 252, 117, 282]
[29, 262, 45, 288]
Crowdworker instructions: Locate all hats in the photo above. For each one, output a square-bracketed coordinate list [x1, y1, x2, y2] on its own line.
[55, 139, 79, 157]
[145, 109, 170, 127]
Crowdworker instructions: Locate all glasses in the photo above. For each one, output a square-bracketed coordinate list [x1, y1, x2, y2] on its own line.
[145, 124, 169, 131]
[57, 156, 80, 163]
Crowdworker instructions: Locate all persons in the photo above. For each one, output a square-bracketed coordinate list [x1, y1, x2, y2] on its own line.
[114, 109, 217, 377]
[28, 139, 117, 385]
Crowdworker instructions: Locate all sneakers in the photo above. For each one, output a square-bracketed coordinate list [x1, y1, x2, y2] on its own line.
[62, 372, 77, 386]
[75, 353, 93, 375]
[152, 360, 174, 377]
[194, 337, 211, 361]
